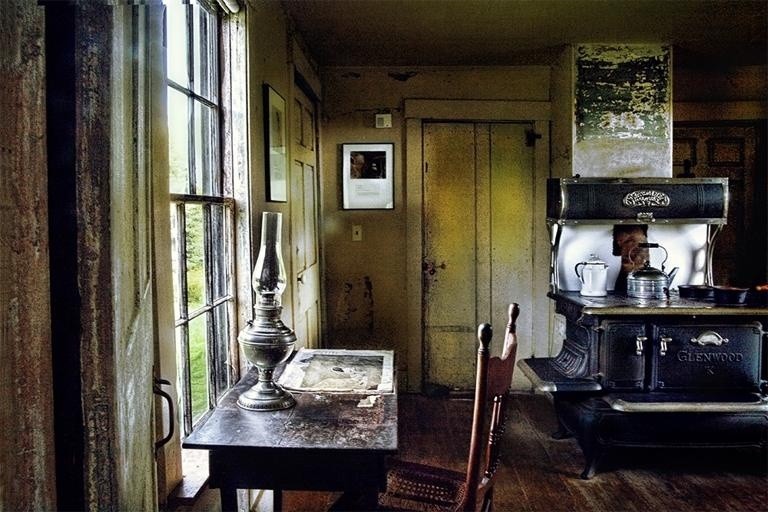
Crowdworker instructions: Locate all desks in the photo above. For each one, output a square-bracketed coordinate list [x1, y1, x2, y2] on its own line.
[182, 348, 396, 512]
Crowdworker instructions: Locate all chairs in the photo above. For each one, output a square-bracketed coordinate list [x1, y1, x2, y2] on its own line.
[374, 303, 521, 510]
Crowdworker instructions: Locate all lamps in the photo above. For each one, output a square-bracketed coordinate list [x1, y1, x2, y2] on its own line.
[237, 213, 296, 410]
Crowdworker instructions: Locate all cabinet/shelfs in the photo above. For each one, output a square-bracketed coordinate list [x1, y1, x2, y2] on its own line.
[546, 177, 729, 295]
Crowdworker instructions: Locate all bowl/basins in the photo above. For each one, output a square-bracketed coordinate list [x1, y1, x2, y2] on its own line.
[678, 285, 712, 298]
[713, 285, 749, 304]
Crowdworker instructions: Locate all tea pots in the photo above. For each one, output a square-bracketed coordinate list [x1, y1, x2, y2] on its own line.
[575, 255, 610, 297]
[629, 243, 679, 299]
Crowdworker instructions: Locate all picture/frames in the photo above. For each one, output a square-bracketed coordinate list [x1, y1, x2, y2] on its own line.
[341, 144, 395, 211]
[264, 84, 290, 202]
